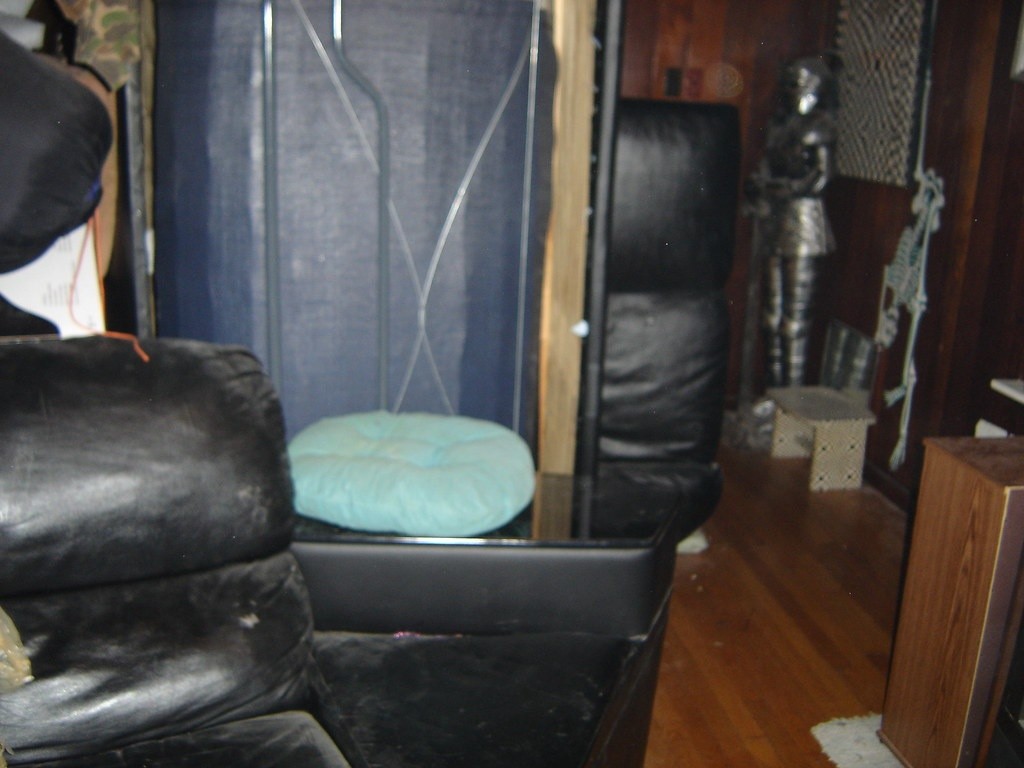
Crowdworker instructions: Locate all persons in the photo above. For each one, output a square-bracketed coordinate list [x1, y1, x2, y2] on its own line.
[753, 57, 832, 417]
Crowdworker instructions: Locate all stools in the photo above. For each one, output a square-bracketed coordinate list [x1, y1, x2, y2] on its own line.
[764, 384, 880, 492]
